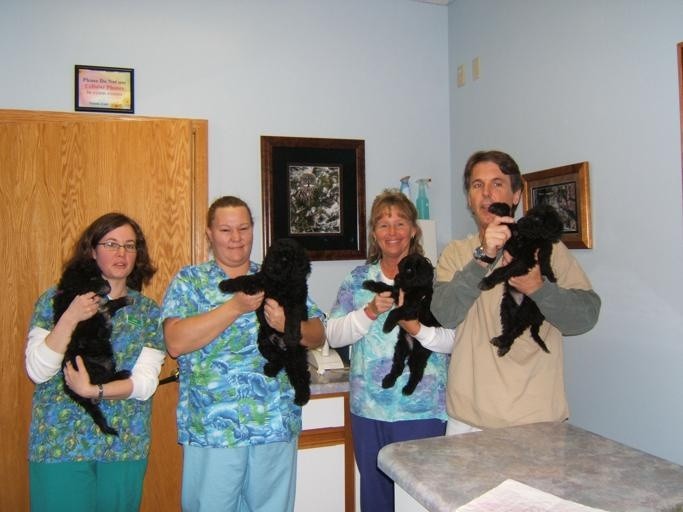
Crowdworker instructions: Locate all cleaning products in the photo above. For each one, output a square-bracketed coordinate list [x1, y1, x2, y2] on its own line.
[414, 177, 432, 220]
[399, 175, 411, 198]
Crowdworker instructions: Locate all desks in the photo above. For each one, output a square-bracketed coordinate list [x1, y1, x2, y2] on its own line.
[377, 420, 682, 512]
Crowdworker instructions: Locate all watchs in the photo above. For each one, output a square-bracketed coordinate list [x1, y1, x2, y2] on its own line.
[473, 247, 496, 264]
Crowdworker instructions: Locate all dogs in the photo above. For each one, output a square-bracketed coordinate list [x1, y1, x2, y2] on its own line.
[361, 252, 443, 397]
[217, 236, 313, 407]
[475, 200, 565, 359]
[51, 255, 135, 438]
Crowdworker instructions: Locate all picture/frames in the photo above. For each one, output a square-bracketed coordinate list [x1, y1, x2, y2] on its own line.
[72, 63, 136, 114]
[518, 160, 593, 250]
[257, 133, 366, 263]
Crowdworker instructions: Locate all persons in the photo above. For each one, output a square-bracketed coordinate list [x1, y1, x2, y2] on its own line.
[162, 196, 327, 512]
[25, 213, 167, 512]
[324, 190, 455, 512]
[430, 151, 601, 436]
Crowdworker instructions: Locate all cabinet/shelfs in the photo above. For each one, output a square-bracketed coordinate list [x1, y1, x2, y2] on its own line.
[288, 366, 365, 511]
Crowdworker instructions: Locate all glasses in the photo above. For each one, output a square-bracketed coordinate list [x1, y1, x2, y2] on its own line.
[95, 241, 137, 252]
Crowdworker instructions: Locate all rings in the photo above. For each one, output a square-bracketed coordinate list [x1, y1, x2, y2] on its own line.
[90, 298, 94, 305]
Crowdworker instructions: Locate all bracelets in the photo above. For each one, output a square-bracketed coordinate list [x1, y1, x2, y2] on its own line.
[364, 306, 377, 321]
[97, 384, 103, 401]
